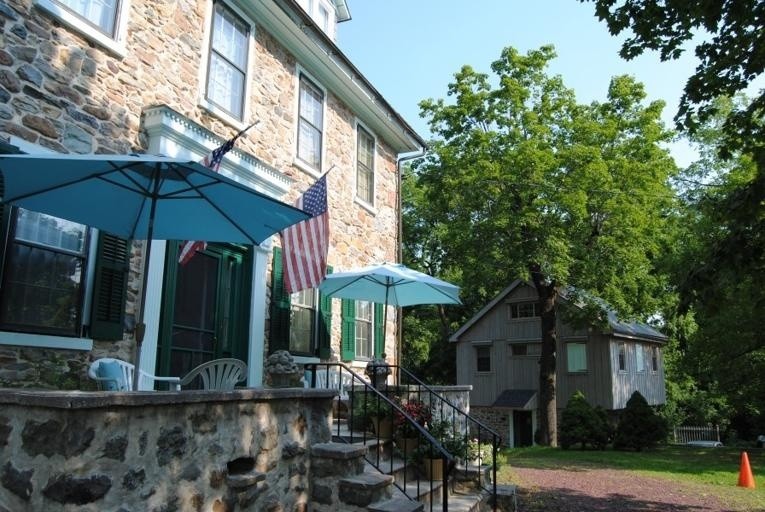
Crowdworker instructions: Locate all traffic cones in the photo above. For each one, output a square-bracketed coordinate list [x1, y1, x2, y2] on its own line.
[737, 451, 755, 488]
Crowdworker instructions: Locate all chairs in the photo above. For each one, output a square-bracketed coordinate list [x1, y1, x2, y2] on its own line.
[300, 370, 360, 401]
[89, 358, 180, 390]
[169, 358, 247, 390]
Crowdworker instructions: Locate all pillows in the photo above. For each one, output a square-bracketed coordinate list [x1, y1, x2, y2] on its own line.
[98, 360, 127, 391]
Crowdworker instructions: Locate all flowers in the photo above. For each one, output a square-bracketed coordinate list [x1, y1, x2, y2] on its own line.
[466, 439, 485, 450]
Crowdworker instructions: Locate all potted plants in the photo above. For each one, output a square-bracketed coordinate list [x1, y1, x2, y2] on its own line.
[348, 394, 472, 481]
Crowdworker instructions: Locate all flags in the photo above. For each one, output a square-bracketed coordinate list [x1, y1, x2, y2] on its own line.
[176, 128, 249, 267]
[278, 175, 331, 294]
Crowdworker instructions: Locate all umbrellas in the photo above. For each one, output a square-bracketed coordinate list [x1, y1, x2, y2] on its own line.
[0, 150, 315, 401]
[314, 260, 464, 358]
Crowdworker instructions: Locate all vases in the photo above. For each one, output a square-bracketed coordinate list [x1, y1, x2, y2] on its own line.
[468, 450, 485, 466]
